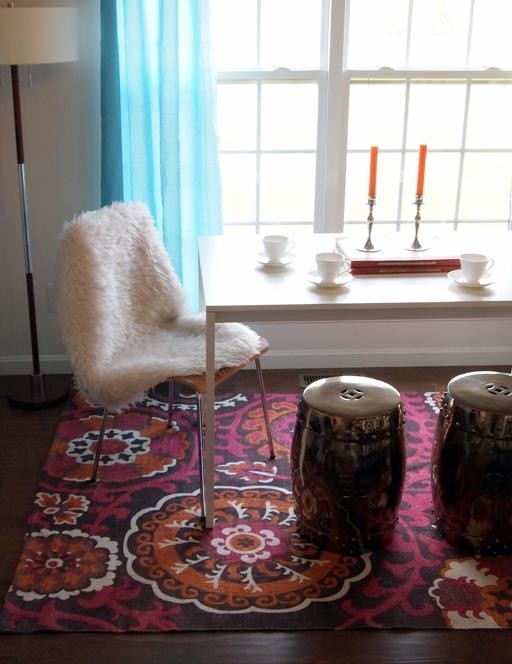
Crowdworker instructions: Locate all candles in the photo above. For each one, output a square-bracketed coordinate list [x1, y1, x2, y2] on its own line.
[368, 144, 378, 199]
[415, 144, 427, 199]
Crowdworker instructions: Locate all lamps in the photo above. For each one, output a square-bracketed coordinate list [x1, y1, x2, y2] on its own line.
[0, 0, 81, 408]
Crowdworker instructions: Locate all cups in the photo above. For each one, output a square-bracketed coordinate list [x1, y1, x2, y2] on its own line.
[458, 254, 494, 283]
[263, 235, 297, 263]
[316, 252, 352, 283]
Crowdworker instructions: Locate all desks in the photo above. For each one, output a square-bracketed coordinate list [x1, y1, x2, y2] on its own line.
[196, 232, 512, 529]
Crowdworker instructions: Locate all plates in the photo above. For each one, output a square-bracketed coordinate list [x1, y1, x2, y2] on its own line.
[446, 269, 497, 289]
[255, 252, 299, 269]
[305, 268, 354, 290]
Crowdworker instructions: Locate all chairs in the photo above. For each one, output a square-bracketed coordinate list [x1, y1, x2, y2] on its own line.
[70, 200, 275, 521]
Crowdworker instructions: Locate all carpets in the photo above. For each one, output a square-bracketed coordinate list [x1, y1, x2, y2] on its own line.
[0, 386, 512, 635]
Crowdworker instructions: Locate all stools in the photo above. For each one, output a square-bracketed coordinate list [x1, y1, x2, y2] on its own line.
[430, 369, 512, 552]
[289, 375, 406, 555]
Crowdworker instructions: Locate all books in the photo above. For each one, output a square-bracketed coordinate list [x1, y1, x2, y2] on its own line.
[334, 233, 462, 268]
[332, 242, 462, 275]
